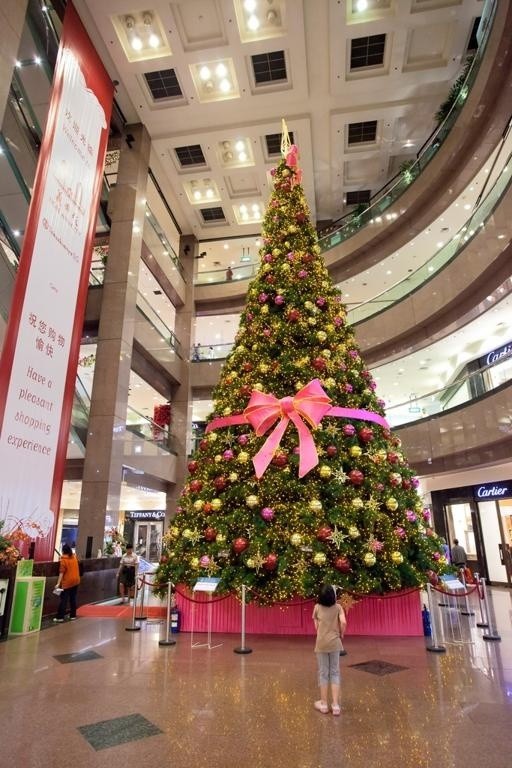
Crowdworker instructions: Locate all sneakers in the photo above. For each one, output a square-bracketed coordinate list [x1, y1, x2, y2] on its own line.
[331, 703, 341, 716]
[53, 616, 76, 622]
[314, 700, 328, 714]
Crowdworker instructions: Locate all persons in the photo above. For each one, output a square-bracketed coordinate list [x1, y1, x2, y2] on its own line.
[226, 266, 234, 280]
[115, 541, 123, 557]
[53, 543, 81, 624]
[115, 543, 140, 604]
[451, 538, 468, 568]
[311, 583, 349, 716]
[440, 536, 451, 566]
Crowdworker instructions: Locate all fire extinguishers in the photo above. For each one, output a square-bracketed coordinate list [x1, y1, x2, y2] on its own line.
[170, 607, 180, 633]
[422, 603, 432, 637]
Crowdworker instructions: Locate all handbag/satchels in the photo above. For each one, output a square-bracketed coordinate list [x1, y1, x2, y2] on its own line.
[340, 647, 347, 656]
[464, 566, 473, 584]
